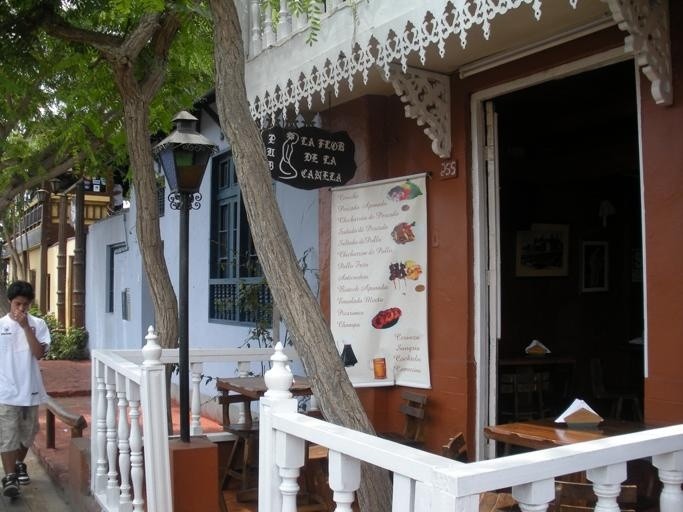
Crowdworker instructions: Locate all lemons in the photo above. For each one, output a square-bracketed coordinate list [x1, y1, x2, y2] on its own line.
[402, 183, 422, 200]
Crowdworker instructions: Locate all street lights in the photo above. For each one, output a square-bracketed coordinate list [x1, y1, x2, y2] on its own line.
[149, 110, 220, 441]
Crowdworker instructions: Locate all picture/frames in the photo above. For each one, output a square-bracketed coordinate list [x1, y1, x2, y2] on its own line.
[516, 230, 569, 277]
[579, 240, 608, 292]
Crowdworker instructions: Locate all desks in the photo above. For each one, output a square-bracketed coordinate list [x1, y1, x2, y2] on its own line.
[498, 356, 576, 421]
[216, 374, 314, 469]
[485, 417, 668, 509]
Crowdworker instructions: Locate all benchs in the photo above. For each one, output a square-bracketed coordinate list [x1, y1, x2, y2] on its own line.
[595, 392, 641, 421]
[221, 411, 322, 492]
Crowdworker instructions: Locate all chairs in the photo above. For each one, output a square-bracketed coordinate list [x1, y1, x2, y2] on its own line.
[549, 481, 637, 512]
[442, 432, 519, 512]
[376, 392, 428, 447]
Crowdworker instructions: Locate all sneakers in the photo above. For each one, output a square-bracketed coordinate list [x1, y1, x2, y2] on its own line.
[2, 462, 31, 497]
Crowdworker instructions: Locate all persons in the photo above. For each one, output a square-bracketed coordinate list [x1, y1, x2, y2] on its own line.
[0, 280, 50, 497]
